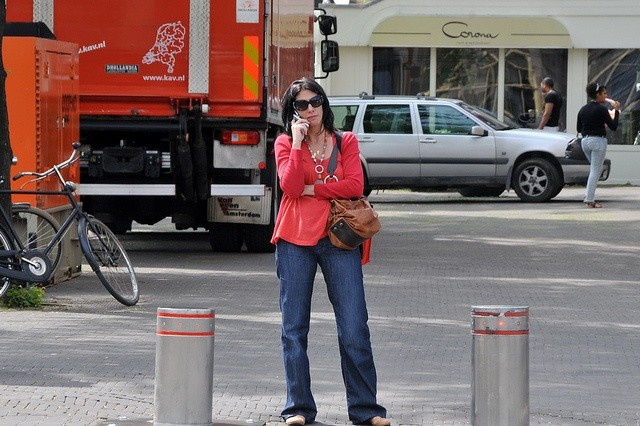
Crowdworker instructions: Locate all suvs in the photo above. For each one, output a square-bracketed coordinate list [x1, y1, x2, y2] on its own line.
[327, 94, 612, 201]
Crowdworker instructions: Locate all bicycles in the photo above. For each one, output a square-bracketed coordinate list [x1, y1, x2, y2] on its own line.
[13, 203, 61, 287]
[0, 140, 140, 309]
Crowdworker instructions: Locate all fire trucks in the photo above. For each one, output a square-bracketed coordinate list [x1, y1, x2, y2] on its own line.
[0, 0, 338, 252]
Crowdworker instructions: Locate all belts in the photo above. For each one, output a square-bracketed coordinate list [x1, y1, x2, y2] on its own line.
[583, 135, 607, 138]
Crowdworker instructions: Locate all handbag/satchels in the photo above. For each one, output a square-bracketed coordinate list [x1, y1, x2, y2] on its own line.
[326, 196, 381, 250]
[565, 133, 587, 160]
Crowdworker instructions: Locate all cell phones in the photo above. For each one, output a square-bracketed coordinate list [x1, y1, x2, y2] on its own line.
[292, 109, 310, 129]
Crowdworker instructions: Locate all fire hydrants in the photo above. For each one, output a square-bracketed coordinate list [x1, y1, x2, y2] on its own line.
[0, 36, 80, 282]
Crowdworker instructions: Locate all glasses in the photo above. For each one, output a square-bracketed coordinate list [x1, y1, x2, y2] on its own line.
[293, 95, 323, 111]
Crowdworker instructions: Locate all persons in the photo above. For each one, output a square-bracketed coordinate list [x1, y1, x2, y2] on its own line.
[577, 84, 621, 208]
[538, 77, 563, 132]
[270, 78, 393, 425]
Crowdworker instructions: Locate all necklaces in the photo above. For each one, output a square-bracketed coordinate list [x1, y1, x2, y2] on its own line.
[304, 129, 328, 184]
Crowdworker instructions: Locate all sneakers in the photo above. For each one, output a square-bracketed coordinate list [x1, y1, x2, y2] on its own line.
[371, 415, 391, 426]
[286, 415, 305, 425]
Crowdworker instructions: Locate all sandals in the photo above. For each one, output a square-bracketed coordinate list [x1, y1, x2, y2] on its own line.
[584, 202, 603, 208]
